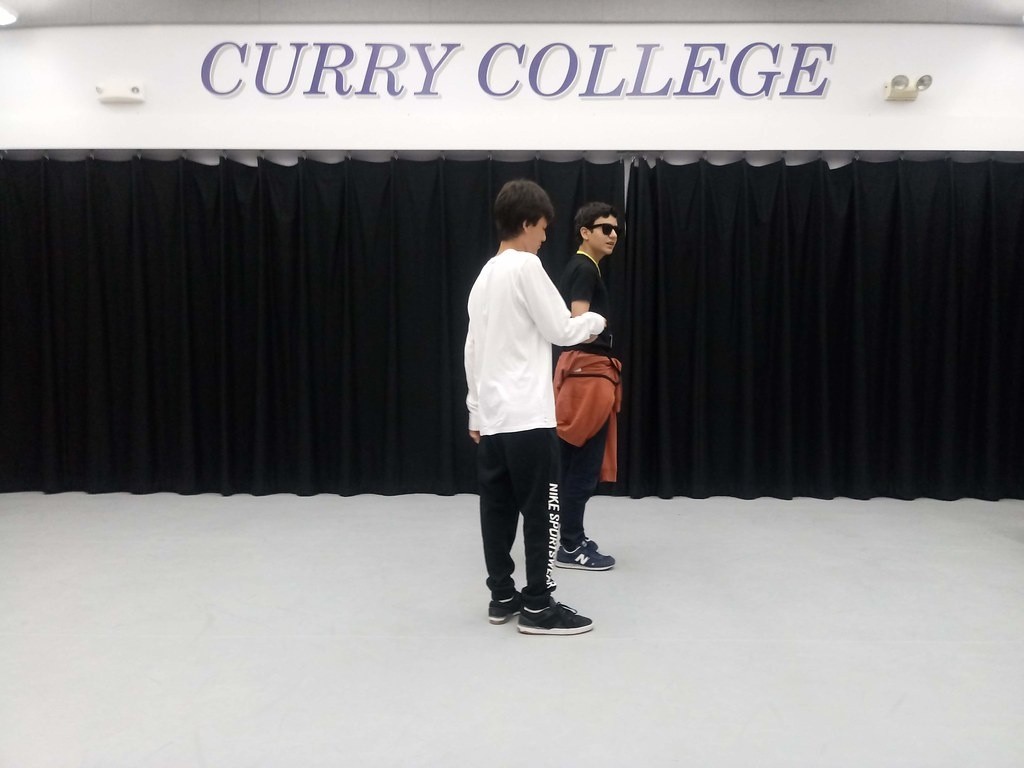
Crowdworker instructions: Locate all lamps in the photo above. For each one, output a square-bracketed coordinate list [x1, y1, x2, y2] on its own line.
[94, 83, 145, 104]
[883, 74, 934, 101]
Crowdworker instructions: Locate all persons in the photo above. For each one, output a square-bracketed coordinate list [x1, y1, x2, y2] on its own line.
[552, 202, 623, 571]
[464, 179, 607, 636]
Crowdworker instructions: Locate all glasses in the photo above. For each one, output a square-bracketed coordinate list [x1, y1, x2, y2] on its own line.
[587, 223, 618, 235]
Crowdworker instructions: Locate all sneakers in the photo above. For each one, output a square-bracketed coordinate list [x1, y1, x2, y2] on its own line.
[489, 589, 522, 625]
[517, 601, 593, 634]
[554, 533, 615, 571]
